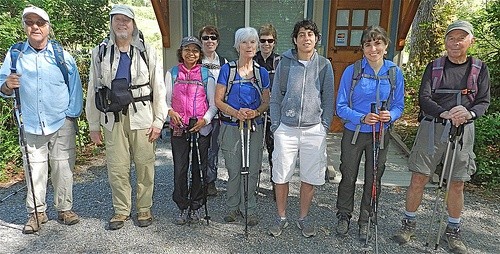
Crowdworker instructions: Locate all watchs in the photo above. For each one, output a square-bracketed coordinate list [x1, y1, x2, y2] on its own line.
[256, 109, 260, 117]
[470, 111, 476, 119]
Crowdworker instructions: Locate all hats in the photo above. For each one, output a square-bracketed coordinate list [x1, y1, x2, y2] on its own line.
[22, 6, 50, 23]
[181, 36, 203, 48]
[445, 21, 474, 36]
[109, 4, 135, 19]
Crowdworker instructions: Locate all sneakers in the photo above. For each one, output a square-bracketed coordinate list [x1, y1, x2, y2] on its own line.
[175, 208, 204, 225]
[23, 211, 48, 233]
[58, 210, 81, 225]
[24, 18, 49, 27]
[267, 217, 288, 236]
[295, 216, 316, 237]
[336, 213, 350, 235]
[394, 218, 416, 243]
[441, 225, 468, 253]
[359, 224, 373, 240]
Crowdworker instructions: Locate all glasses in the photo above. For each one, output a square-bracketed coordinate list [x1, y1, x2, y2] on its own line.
[259, 38, 275, 43]
[202, 36, 219, 40]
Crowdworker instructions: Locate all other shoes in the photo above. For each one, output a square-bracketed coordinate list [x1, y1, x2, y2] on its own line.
[247, 215, 258, 226]
[224, 210, 237, 222]
[138, 210, 154, 226]
[207, 181, 217, 196]
[109, 212, 131, 230]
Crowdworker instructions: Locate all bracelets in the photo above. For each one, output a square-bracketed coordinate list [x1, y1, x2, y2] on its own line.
[5, 82, 11, 90]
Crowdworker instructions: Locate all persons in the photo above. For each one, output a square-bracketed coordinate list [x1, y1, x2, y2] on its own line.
[393, 20, 490, 254]
[270, 19, 334, 238]
[164, 37, 219, 225]
[253, 24, 283, 201]
[0, 7, 84, 232]
[214, 27, 271, 226]
[335, 25, 405, 242]
[86, 5, 168, 229]
[199, 24, 231, 196]
